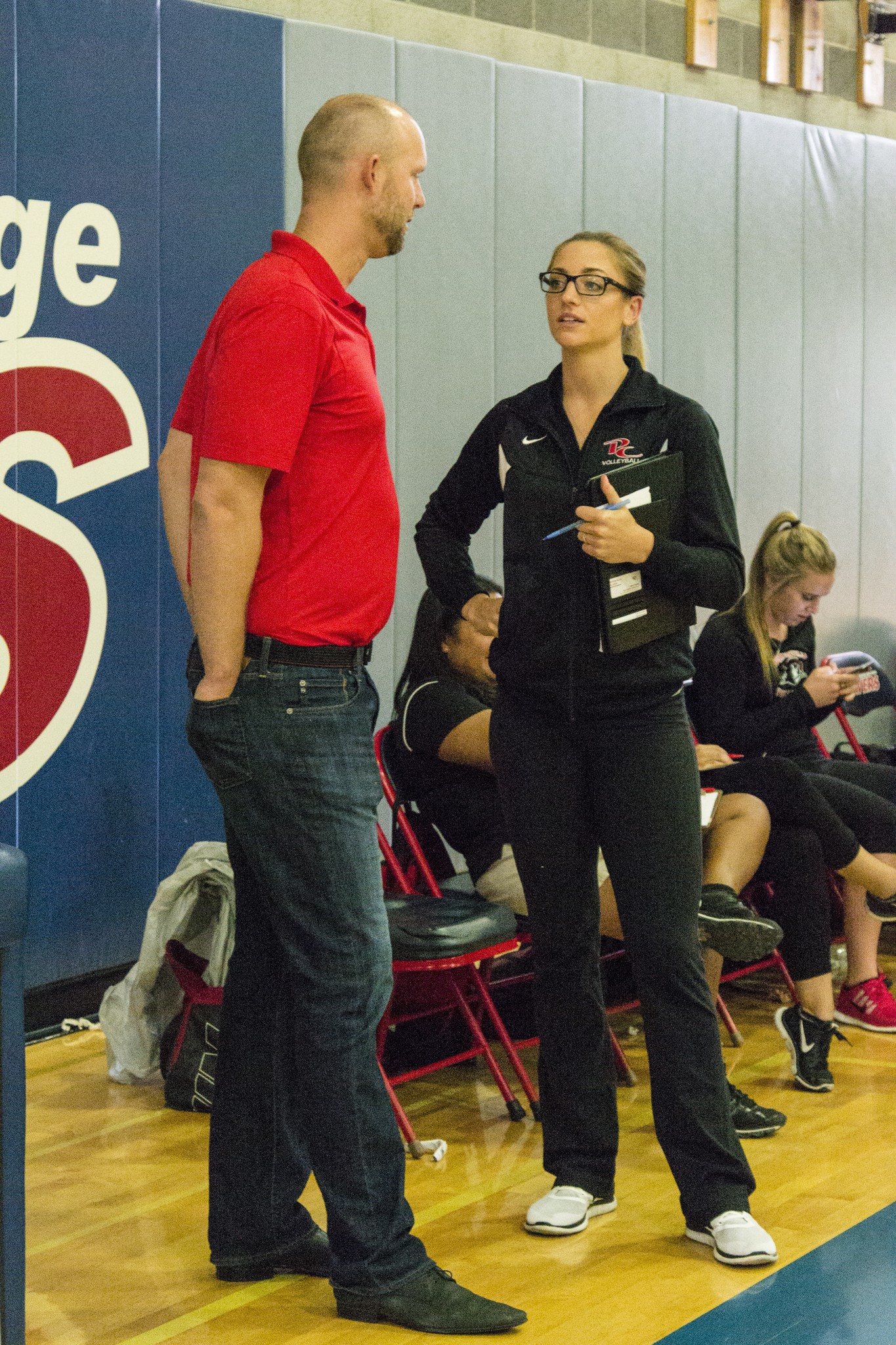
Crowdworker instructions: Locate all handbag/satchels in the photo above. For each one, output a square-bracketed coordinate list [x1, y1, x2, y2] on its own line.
[153, 939, 226, 1110]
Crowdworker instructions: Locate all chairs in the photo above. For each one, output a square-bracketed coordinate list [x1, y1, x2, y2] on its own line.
[371, 652, 896, 1159]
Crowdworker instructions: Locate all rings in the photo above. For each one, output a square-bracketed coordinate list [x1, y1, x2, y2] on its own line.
[583, 533, 589, 545]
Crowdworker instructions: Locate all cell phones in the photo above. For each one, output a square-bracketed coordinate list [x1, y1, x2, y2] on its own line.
[849, 660, 875, 674]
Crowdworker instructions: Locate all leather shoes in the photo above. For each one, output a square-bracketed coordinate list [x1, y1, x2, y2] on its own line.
[214, 1226, 330, 1281]
[330, 1261, 528, 1333]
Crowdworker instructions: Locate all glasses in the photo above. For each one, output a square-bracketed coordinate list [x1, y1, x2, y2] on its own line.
[539, 270, 642, 301]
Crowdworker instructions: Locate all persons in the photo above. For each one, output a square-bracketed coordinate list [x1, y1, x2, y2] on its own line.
[414, 229, 780, 1266]
[687, 509, 896, 1033]
[390, 579, 788, 1137]
[692, 743, 895, 1092]
[157, 95, 532, 1336]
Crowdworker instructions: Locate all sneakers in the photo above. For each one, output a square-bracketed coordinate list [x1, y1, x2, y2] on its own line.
[773, 1000, 850, 1090]
[834, 967, 896, 1034]
[522, 1183, 617, 1236]
[725, 1080, 786, 1137]
[696, 887, 782, 962]
[865, 887, 896, 921]
[684, 1209, 780, 1268]
